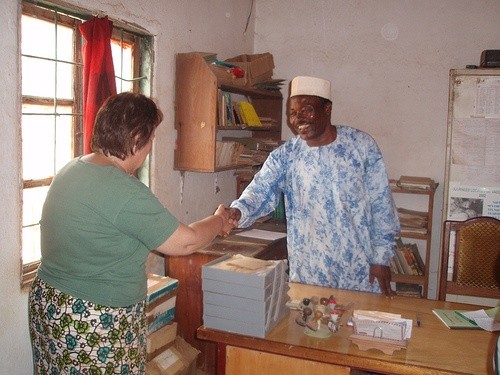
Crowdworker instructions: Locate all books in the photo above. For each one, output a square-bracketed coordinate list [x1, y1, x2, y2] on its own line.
[432, 308, 484, 330]
[267, 193, 286, 219]
[218, 87, 277, 166]
[390, 176, 440, 277]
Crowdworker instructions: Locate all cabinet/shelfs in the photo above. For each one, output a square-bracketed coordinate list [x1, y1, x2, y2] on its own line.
[164, 222, 288, 375]
[391, 188, 435, 298]
[173, 53, 284, 173]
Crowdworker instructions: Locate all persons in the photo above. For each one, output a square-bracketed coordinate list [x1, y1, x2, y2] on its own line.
[27, 91, 229, 375]
[217, 76, 399, 293]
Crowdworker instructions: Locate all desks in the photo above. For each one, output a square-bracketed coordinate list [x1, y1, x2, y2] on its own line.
[196, 280, 500, 375]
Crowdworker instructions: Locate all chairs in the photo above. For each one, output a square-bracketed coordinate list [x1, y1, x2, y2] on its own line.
[439, 215, 500, 302]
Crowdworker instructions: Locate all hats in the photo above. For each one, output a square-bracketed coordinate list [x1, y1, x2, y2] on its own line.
[290, 76, 332, 102]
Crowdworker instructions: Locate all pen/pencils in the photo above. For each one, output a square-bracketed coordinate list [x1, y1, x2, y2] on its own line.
[454, 310, 477, 325]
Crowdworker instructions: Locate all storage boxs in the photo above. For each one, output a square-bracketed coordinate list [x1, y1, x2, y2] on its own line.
[222, 53, 275, 88]
[141, 272, 202, 375]
